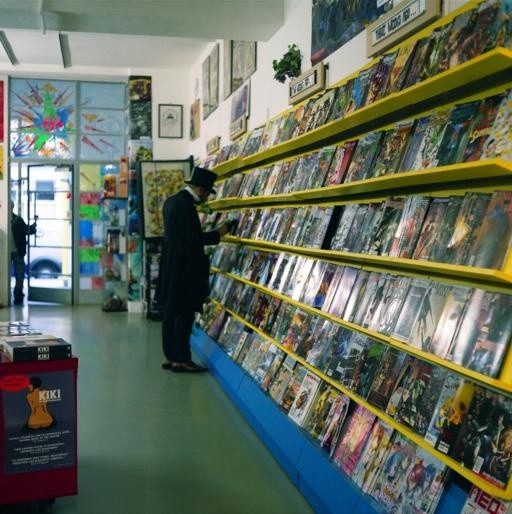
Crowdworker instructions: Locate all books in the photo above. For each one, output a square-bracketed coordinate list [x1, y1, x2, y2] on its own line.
[0, 320, 43, 346]
[4, 349, 73, 361]
[3, 336, 73, 353]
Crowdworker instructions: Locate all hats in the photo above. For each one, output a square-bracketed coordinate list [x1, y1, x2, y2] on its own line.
[185, 166, 218, 195]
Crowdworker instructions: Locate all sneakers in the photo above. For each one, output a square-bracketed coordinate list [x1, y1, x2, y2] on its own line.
[14, 297, 23, 307]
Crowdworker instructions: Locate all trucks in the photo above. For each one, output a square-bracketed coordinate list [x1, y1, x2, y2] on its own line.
[11, 166, 71, 279]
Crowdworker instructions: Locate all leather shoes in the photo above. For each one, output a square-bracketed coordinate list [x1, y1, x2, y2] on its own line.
[161, 360, 208, 374]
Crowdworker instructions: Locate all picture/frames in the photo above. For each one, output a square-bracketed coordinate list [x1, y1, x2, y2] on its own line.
[158, 102, 183, 139]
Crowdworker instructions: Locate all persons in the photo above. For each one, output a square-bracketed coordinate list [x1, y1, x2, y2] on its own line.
[159, 164, 234, 374]
[8, 198, 38, 306]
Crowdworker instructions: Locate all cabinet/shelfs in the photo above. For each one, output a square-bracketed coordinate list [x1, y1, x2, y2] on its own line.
[188, 43, 511, 502]
